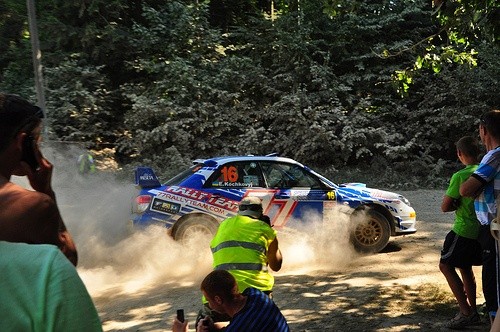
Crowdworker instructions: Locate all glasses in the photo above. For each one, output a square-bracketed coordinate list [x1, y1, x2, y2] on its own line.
[479, 114, 489, 129]
[238, 204, 263, 212]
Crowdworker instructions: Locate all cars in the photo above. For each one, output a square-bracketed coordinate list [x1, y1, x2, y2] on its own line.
[130, 154, 418, 256]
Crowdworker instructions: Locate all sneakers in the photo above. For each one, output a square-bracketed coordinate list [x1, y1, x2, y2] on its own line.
[444, 311, 478, 329]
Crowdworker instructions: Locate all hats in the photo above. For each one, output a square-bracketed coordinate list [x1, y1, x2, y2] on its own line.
[238, 197, 262, 219]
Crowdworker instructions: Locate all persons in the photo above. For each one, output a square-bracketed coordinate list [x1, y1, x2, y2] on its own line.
[202, 196, 283, 322]
[0, 91, 79, 271]
[1, 239, 103, 332]
[438, 136, 485, 330]
[173, 269, 289, 332]
[459, 109, 500, 326]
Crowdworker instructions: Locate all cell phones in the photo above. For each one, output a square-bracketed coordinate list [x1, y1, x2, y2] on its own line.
[177, 309, 184, 324]
[23, 135, 40, 170]
